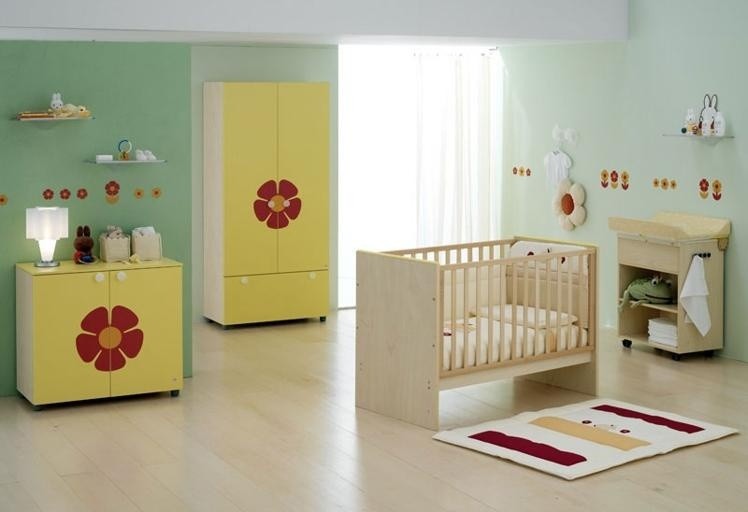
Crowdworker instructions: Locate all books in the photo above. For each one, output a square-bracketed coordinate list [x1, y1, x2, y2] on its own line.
[17, 112, 49, 120]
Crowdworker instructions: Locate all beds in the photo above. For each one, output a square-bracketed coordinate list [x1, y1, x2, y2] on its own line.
[355, 235, 599, 430]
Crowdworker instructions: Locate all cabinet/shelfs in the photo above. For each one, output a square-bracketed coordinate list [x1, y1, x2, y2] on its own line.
[14, 257, 184, 412]
[200, 81, 331, 329]
[615, 234, 724, 362]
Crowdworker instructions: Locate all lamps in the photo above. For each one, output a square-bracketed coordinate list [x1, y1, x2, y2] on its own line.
[24, 205, 70, 268]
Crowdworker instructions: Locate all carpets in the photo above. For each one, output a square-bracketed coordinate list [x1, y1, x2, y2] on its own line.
[432, 397, 741, 482]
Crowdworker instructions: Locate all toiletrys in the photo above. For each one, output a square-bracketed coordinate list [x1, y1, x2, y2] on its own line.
[714, 112, 726, 137]
[702, 120, 710, 136]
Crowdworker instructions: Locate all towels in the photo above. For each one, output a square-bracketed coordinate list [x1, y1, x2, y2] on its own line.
[678, 255, 712, 336]
[647, 316, 678, 349]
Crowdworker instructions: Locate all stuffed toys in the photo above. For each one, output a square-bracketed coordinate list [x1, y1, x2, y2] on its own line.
[48, 93, 65, 118]
[64, 103, 88, 117]
[619, 276, 673, 310]
[73, 225, 96, 264]
[684, 108, 698, 135]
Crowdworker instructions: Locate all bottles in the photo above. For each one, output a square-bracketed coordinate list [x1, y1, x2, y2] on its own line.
[702, 112, 726, 137]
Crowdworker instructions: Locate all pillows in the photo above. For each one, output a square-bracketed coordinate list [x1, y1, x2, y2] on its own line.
[470, 304, 578, 329]
